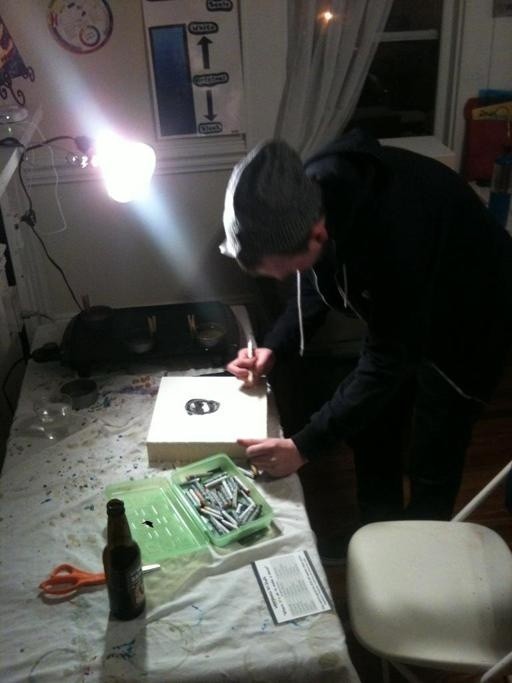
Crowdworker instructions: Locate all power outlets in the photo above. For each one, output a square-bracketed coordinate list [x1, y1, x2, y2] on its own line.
[3, 288, 24, 333]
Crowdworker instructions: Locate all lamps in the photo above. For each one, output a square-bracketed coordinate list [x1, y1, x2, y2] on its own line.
[16, 135, 158, 369]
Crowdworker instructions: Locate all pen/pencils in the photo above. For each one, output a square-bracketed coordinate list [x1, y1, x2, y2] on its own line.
[180, 472, 262, 536]
[247, 335, 255, 387]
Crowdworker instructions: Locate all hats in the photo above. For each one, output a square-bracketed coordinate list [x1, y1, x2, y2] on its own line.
[223, 141, 315, 260]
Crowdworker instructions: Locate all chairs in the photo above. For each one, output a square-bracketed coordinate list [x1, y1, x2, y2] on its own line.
[346, 459, 512, 683]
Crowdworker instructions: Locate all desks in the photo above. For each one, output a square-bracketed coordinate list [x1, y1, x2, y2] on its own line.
[0, 307, 361, 682]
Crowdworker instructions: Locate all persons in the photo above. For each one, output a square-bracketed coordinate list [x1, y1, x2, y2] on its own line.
[218, 133, 512, 568]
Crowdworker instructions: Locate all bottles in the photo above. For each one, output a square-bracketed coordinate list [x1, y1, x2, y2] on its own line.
[101, 497, 146, 621]
[487, 142, 511, 228]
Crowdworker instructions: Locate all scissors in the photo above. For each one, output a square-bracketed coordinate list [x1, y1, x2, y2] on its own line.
[38, 563, 160, 595]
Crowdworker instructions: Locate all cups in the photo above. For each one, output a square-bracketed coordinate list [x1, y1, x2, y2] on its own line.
[32, 391, 75, 441]
[79, 304, 114, 338]
[191, 321, 227, 353]
